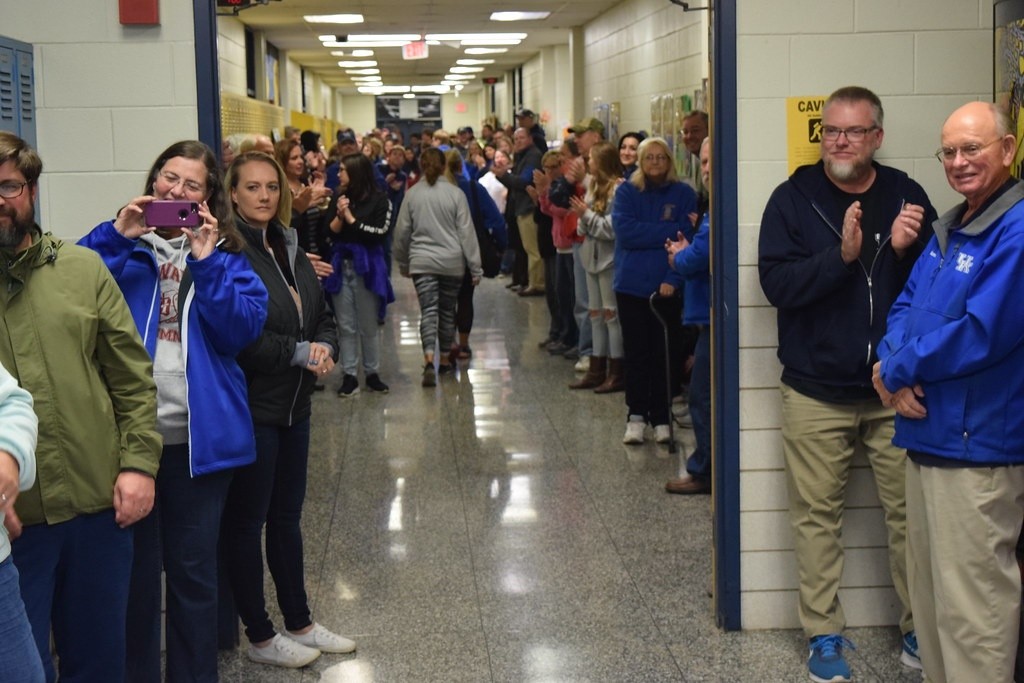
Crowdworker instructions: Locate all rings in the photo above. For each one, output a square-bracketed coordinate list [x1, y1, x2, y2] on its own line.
[1, 494, 7, 503]
[323, 369, 327, 373]
[140, 509, 146, 512]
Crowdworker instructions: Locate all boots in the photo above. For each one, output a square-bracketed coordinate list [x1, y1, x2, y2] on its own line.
[594, 357, 627, 393]
[569, 357, 606, 388]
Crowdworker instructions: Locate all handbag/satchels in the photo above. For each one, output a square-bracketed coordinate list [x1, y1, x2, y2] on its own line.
[470, 179, 500, 278]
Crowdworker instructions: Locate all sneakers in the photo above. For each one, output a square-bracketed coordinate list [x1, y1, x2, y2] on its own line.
[284, 621, 356, 654]
[653, 425, 670, 443]
[365, 373, 389, 394]
[248, 634, 320, 667]
[337, 373, 360, 396]
[899, 630, 925, 668]
[808, 629, 856, 683]
[624, 414, 647, 444]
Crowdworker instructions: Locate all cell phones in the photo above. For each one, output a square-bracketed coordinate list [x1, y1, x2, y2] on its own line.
[143, 200, 200, 228]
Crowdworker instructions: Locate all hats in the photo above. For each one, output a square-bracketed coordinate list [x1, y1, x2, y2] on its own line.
[336, 128, 357, 144]
[566, 116, 605, 135]
[514, 109, 535, 119]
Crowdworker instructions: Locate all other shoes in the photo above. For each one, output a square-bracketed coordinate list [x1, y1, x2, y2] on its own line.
[535, 335, 579, 359]
[504, 281, 542, 296]
[422, 363, 435, 388]
[438, 363, 450, 375]
[451, 345, 472, 359]
[665, 473, 712, 494]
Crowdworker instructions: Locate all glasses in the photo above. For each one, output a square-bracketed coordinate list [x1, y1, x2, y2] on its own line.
[543, 162, 562, 172]
[820, 122, 876, 144]
[0, 179, 30, 201]
[158, 169, 209, 197]
[934, 138, 1002, 163]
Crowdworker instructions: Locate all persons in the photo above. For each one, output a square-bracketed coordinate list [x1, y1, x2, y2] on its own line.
[0, 126, 163, 683]
[73, 139, 270, 683]
[217, 152, 356, 668]
[872, 102, 1024, 683]
[0, 360, 45, 683]
[222, 110, 713, 496]
[758, 87, 938, 683]
